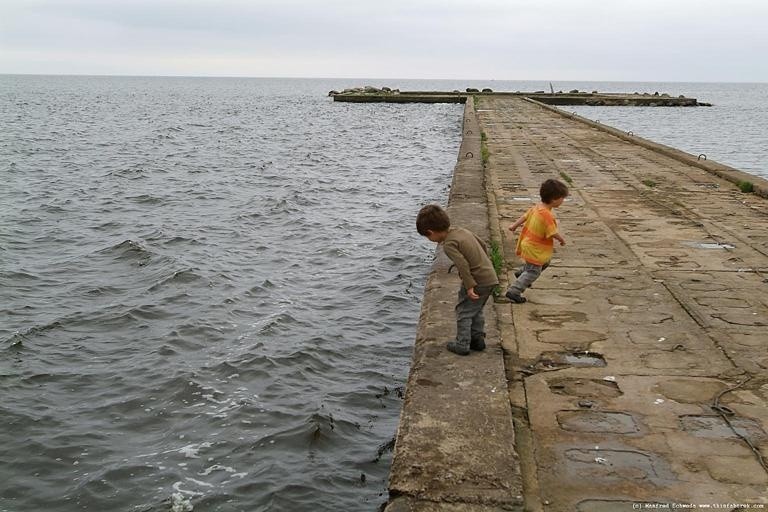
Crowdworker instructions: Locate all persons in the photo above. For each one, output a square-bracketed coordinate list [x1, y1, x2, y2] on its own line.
[415, 202, 500, 356]
[505, 178, 570, 305]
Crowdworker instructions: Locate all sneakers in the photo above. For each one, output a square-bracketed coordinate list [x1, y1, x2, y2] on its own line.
[506, 291, 526, 302]
[471, 335, 485, 350]
[447, 341, 470, 355]
[514, 269, 531, 288]
